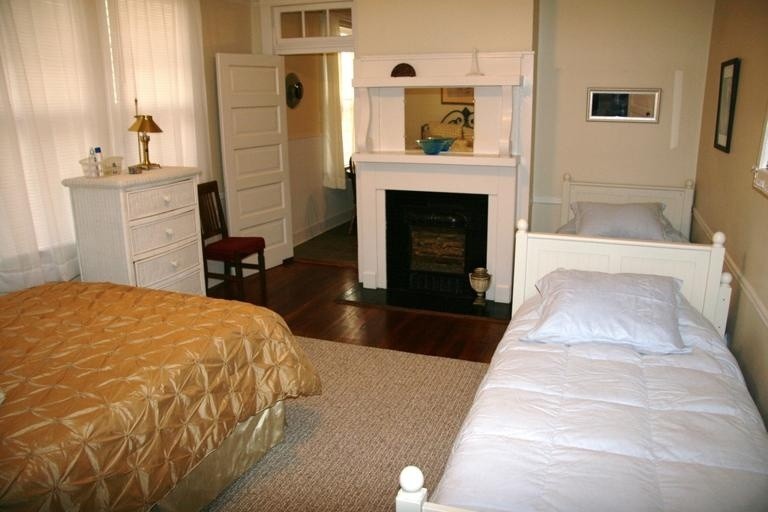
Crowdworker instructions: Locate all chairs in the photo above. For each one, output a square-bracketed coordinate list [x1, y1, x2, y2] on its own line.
[198, 181, 267, 306]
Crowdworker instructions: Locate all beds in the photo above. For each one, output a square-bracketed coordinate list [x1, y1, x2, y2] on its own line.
[557, 173, 733, 338]
[395, 219, 764, 512]
[0, 280, 321, 512]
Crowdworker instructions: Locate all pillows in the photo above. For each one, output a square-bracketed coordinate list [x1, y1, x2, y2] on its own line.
[571, 202, 666, 240]
[519, 270, 693, 355]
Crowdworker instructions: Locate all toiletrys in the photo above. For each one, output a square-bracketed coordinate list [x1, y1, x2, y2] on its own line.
[95, 147, 104, 179]
[88, 146, 99, 179]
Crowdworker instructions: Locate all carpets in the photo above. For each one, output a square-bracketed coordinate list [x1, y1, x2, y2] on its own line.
[294, 218, 358, 264]
[196, 336, 488, 512]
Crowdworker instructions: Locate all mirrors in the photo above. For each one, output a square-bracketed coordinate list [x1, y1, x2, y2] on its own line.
[404, 87, 475, 155]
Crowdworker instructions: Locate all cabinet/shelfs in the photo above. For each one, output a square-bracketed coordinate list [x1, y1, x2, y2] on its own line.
[62, 166, 206, 297]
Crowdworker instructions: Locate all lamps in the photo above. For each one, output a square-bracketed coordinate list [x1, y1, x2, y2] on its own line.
[128, 115, 163, 171]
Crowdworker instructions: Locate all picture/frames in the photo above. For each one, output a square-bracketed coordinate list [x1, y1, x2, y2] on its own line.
[751, 122, 768, 199]
[584, 86, 661, 122]
[713, 57, 740, 153]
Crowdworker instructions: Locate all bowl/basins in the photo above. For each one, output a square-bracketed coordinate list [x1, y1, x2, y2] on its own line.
[433, 137, 457, 151]
[416, 138, 447, 155]
